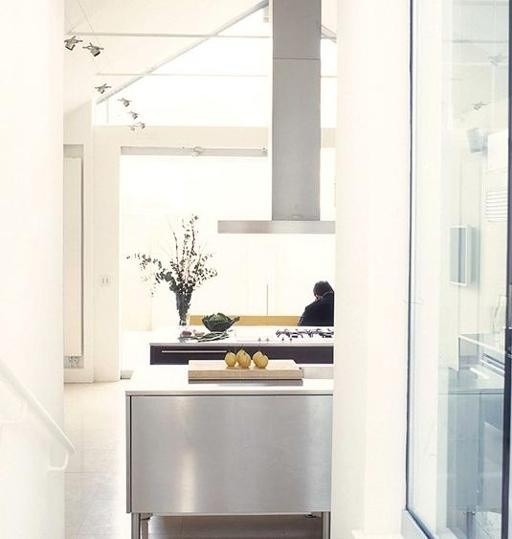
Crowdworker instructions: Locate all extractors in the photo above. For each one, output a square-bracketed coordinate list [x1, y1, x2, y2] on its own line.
[216, 0, 335, 235]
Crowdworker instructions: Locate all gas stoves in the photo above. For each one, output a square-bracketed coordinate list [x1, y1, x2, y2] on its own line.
[275, 325, 333, 341]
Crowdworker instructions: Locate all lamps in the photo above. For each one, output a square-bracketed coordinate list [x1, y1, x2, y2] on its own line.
[64, 33, 80, 53]
[119, 97, 146, 129]
[93, 82, 111, 94]
[83, 41, 106, 59]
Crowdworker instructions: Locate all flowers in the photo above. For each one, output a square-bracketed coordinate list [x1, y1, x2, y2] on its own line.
[124, 211, 220, 327]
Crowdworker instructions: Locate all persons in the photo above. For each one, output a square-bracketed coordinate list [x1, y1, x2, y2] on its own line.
[298, 281, 334, 326]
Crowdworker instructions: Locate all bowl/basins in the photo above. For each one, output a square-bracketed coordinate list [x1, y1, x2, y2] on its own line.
[202, 318, 234, 331]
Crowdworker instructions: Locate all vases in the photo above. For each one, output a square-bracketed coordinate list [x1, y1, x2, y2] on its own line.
[170, 289, 195, 336]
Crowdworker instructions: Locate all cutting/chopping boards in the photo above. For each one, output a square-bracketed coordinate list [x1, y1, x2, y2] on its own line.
[187, 360, 302, 380]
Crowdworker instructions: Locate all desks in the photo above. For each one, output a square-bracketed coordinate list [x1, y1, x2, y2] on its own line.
[147, 324, 335, 367]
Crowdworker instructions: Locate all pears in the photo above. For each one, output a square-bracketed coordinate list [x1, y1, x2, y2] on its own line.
[225, 349, 268, 368]
